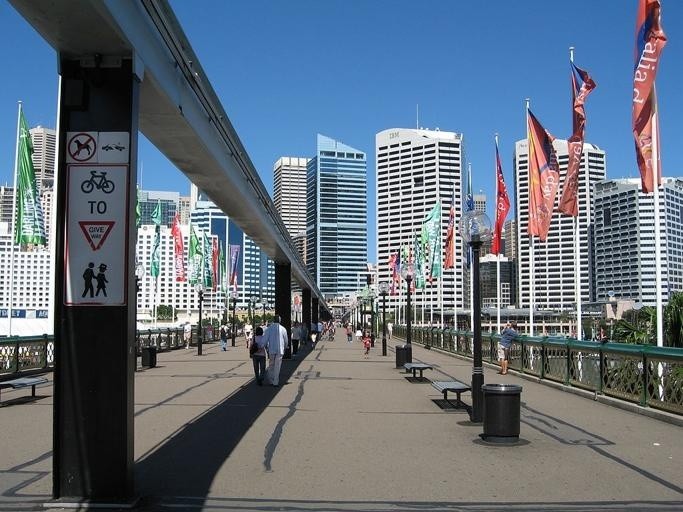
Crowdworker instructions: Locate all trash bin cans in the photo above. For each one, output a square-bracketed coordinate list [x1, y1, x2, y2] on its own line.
[395, 344, 412, 369]
[479, 384, 522, 443]
[141, 345, 157, 368]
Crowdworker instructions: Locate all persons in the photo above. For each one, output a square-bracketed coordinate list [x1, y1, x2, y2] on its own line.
[183, 318, 395, 386]
[499, 321, 519, 376]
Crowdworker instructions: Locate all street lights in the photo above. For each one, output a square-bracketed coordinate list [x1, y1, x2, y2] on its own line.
[261, 299, 268, 325]
[250, 295, 257, 342]
[230, 292, 239, 348]
[349, 291, 377, 348]
[193, 282, 206, 355]
[401, 263, 417, 373]
[378, 280, 389, 357]
[457, 209, 493, 421]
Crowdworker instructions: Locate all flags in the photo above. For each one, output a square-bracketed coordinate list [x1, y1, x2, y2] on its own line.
[524, 106, 560, 242]
[559, 58, 596, 215]
[136, 183, 228, 293]
[294, 288, 301, 314]
[388, 172, 476, 297]
[12, 108, 48, 246]
[631, 0, 673, 193]
[488, 141, 510, 257]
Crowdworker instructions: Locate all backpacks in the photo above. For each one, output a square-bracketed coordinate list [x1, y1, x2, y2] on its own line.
[220, 326, 226, 338]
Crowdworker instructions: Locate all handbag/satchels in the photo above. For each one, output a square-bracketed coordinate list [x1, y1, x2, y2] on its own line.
[250, 343, 258, 354]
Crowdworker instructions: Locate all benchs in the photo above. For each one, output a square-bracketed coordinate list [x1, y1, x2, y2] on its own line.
[403, 362, 433, 384]
[431, 380, 473, 410]
[1, 374, 47, 405]
